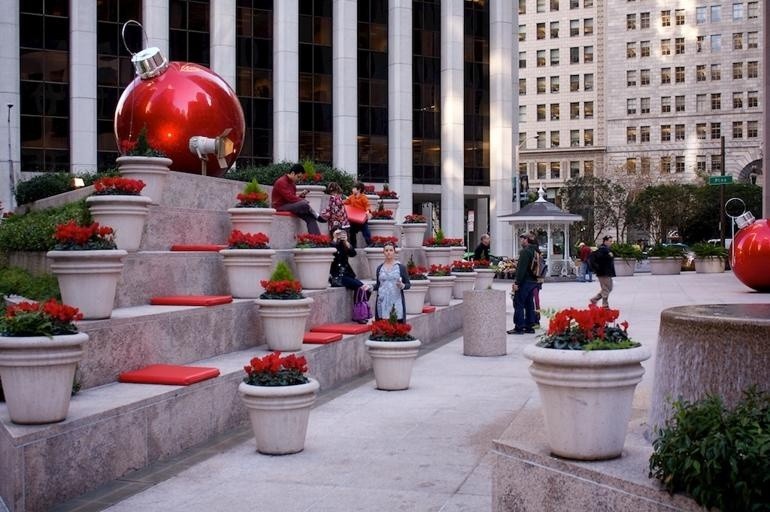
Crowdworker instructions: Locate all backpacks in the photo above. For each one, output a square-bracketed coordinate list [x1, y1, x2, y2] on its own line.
[587, 250, 600, 272]
[532, 249, 548, 283]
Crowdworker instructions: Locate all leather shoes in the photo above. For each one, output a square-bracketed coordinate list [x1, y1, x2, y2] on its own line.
[313, 213, 328, 224]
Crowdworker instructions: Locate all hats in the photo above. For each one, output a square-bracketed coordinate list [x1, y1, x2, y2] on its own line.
[578, 242, 585, 248]
[520, 233, 534, 241]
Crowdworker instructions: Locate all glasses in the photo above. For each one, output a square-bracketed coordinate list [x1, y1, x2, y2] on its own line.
[296, 174, 302, 179]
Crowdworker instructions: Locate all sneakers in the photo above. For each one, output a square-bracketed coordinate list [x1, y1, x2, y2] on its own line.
[524, 326, 536, 334]
[507, 328, 524, 335]
[590, 299, 596, 304]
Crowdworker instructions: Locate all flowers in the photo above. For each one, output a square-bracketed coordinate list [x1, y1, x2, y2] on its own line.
[424, 232, 445, 247]
[372, 210, 395, 219]
[364, 184, 376, 196]
[242, 353, 308, 384]
[378, 183, 398, 198]
[428, 259, 451, 277]
[475, 259, 493, 269]
[402, 254, 430, 280]
[235, 178, 270, 208]
[404, 215, 425, 223]
[0, 299, 84, 336]
[451, 259, 474, 272]
[54, 219, 118, 249]
[446, 238, 464, 247]
[536, 305, 641, 349]
[260, 261, 305, 300]
[294, 232, 331, 248]
[300, 173, 322, 184]
[93, 177, 147, 196]
[123, 129, 168, 158]
[370, 302, 416, 341]
[227, 227, 271, 249]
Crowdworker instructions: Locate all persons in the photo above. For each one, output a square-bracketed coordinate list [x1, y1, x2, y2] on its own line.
[474, 234, 491, 261]
[343, 182, 372, 248]
[578, 242, 594, 281]
[329, 227, 371, 324]
[363, 241, 411, 325]
[590, 235, 616, 307]
[319, 182, 351, 242]
[271, 164, 326, 237]
[637, 240, 642, 247]
[533, 242, 541, 328]
[530, 231, 539, 246]
[506, 233, 536, 334]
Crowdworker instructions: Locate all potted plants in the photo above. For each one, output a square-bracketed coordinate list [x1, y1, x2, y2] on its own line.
[610, 243, 644, 276]
[648, 244, 686, 275]
[692, 240, 730, 274]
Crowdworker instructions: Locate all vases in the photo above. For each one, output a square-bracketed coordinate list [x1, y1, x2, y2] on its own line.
[219, 249, 276, 298]
[522, 340, 651, 461]
[364, 248, 401, 281]
[294, 185, 326, 215]
[365, 340, 421, 391]
[116, 157, 172, 207]
[256, 297, 315, 351]
[48, 249, 128, 318]
[294, 248, 337, 288]
[423, 249, 451, 267]
[87, 196, 153, 252]
[365, 196, 380, 217]
[0, 333, 89, 424]
[472, 269, 496, 290]
[226, 207, 277, 243]
[368, 220, 397, 237]
[426, 276, 457, 306]
[451, 272, 477, 298]
[450, 247, 467, 261]
[403, 280, 431, 314]
[237, 378, 320, 455]
[382, 199, 400, 216]
[399, 223, 428, 247]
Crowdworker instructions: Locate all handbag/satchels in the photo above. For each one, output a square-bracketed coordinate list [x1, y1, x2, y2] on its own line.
[329, 265, 345, 288]
[352, 287, 374, 321]
